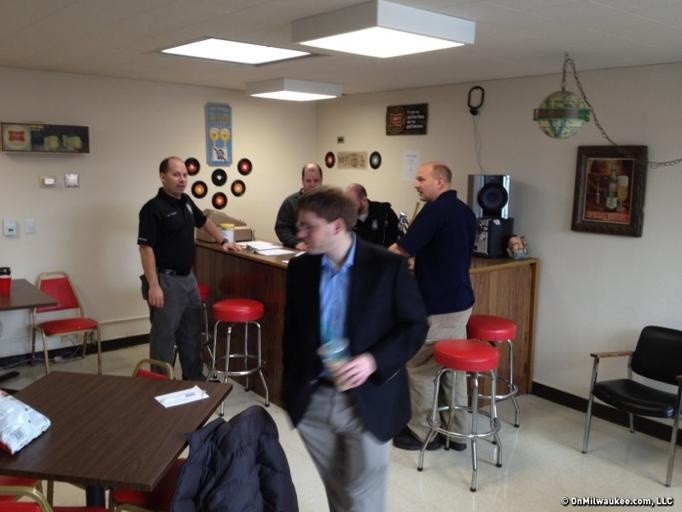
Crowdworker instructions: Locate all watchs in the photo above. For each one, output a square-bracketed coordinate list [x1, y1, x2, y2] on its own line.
[221, 238, 228, 245]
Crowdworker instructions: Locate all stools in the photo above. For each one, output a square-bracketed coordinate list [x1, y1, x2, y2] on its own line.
[466, 313, 521, 445]
[171, 283, 216, 373]
[205, 297, 272, 418]
[416, 339, 505, 494]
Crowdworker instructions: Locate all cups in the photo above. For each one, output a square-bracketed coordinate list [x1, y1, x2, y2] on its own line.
[317, 339, 355, 392]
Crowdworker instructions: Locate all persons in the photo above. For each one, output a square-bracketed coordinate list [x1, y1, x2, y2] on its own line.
[280, 185, 431, 512]
[137, 157, 243, 381]
[344, 183, 400, 250]
[273, 163, 323, 251]
[385, 162, 477, 451]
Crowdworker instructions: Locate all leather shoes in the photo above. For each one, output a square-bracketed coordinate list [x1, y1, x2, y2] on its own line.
[394, 429, 466, 451]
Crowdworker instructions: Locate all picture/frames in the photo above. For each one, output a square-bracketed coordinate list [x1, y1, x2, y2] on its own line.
[571, 144, 648, 239]
[0, 121, 91, 154]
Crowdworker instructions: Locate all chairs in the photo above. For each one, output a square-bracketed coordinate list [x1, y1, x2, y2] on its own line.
[131, 357, 178, 382]
[1, 473, 108, 512]
[582, 324, 681, 490]
[106, 406, 277, 512]
[29, 270, 104, 377]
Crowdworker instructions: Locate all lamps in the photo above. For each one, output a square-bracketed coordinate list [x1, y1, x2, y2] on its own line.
[288, 0, 478, 61]
[243, 78, 342, 103]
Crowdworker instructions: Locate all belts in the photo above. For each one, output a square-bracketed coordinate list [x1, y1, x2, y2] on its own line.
[157, 267, 191, 276]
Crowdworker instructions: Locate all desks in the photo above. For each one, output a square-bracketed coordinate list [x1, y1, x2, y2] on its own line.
[0, 369, 232, 510]
[0, 278, 57, 396]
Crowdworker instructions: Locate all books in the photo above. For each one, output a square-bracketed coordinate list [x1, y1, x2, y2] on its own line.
[236, 240, 295, 256]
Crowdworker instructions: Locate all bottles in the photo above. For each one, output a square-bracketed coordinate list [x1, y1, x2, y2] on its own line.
[219, 222, 234, 242]
[1, 267, 11, 296]
